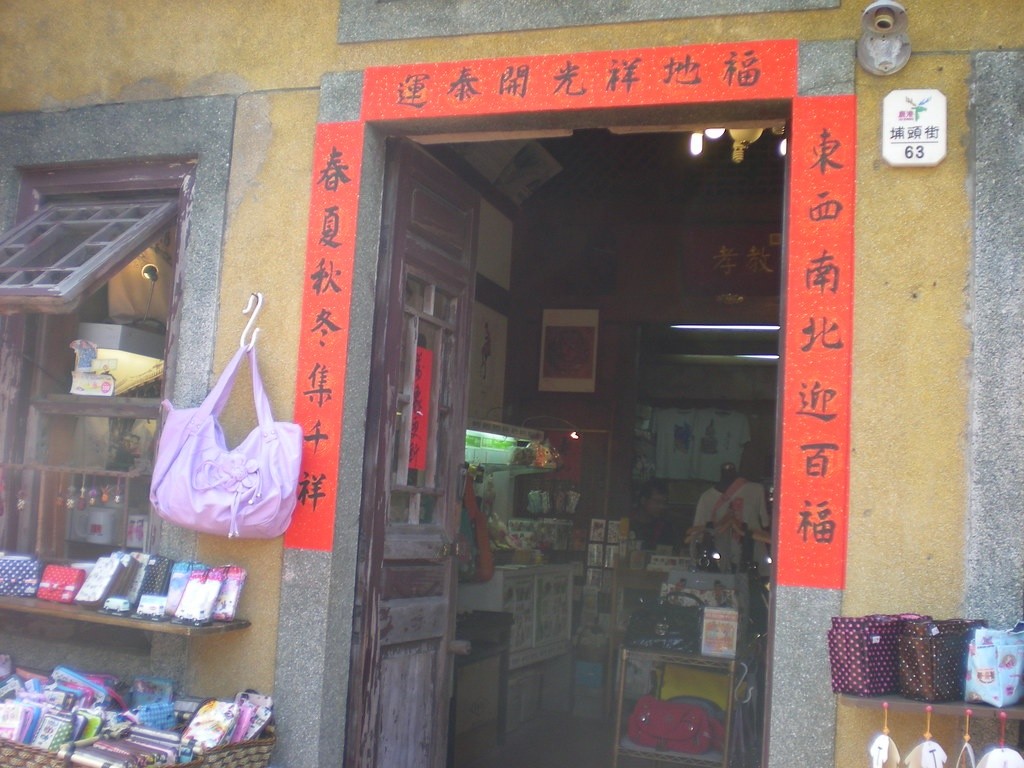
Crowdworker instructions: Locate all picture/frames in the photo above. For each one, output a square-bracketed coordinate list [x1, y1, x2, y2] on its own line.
[535, 302, 603, 402]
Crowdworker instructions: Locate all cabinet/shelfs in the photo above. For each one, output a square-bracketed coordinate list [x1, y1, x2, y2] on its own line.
[612, 633, 767, 767]
[0, 593, 274, 768]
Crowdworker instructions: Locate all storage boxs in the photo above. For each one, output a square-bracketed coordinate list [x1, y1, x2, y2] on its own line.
[452, 520, 573, 737]
[701, 608, 738, 658]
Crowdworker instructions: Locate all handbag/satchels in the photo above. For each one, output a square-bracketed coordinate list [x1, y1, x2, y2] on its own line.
[626, 591, 706, 654]
[627, 694, 724, 756]
[652, 660, 748, 715]
[149, 344, 303, 540]
[965, 620, 1024, 708]
[457, 473, 495, 584]
[827, 611, 933, 698]
[898, 618, 990, 703]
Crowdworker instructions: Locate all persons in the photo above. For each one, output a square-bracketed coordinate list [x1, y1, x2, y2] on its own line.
[693, 463, 771, 578]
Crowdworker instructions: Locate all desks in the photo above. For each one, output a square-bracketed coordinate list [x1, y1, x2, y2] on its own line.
[448, 611, 516, 767]
